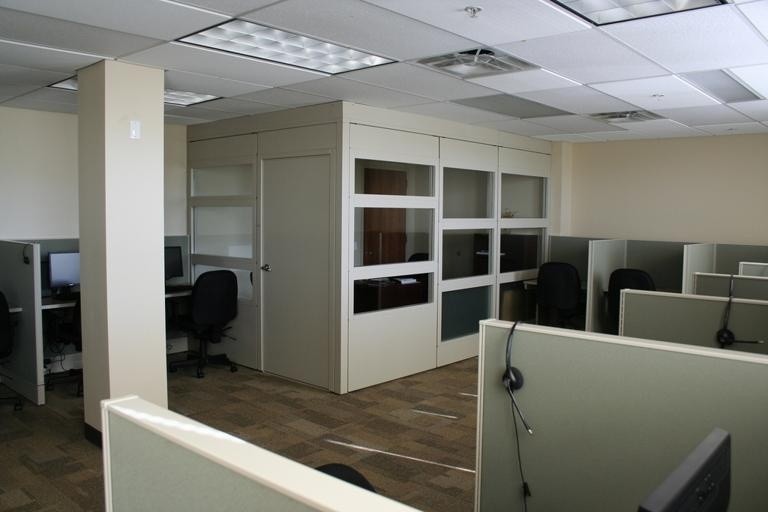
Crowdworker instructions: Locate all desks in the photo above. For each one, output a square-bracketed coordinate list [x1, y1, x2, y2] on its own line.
[354, 277, 422, 315]
[0, 235, 193, 406]
[522, 234, 624, 334]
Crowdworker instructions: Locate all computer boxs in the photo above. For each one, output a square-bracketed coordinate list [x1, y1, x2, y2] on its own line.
[166, 321, 188, 355]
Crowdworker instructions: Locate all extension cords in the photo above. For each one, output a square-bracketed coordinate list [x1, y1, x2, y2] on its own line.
[44, 361, 54, 371]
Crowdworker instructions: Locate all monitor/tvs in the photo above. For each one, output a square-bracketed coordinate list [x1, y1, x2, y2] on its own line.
[165, 246, 184, 287]
[48, 251, 80, 293]
[639, 428, 731, 512]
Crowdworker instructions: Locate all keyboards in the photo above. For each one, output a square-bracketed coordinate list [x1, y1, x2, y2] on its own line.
[52, 294, 80, 301]
[165, 286, 192, 294]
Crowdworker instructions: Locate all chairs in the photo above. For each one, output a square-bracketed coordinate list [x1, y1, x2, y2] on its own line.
[168, 268, 240, 379]
[606, 266, 656, 337]
[407, 252, 431, 279]
[536, 260, 586, 330]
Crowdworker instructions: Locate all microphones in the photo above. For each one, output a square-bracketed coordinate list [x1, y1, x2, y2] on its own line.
[734, 341, 764, 344]
[507, 386, 533, 436]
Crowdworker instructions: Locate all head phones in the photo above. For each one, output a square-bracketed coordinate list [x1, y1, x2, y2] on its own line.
[716, 296, 735, 346]
[503, 322, 523, 390]
[23, 243, 30, 264]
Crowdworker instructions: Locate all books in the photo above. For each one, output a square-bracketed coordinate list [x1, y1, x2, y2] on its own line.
[394, 277, 417, 284]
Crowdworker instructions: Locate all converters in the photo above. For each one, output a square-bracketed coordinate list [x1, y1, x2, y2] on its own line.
[44, 359, 51, 364]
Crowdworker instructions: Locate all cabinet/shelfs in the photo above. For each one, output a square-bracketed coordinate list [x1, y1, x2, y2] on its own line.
[470, 231, 540, 289]
